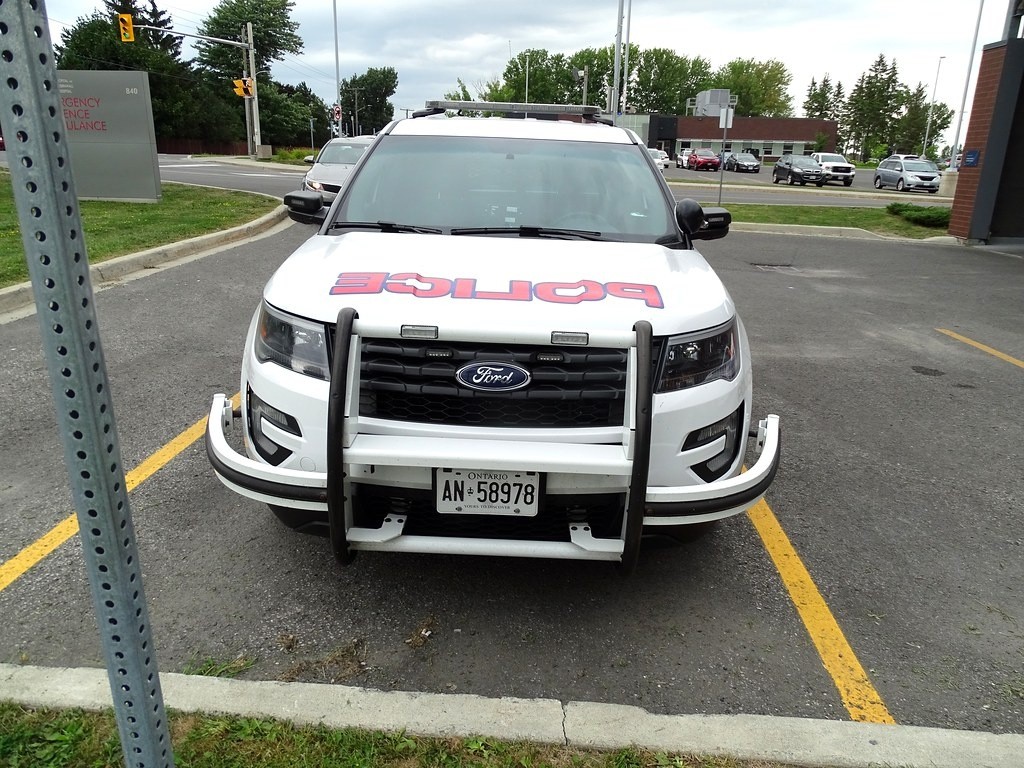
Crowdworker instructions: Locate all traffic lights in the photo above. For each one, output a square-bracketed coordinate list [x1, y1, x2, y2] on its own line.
[233, 80, 245, 96]
[119, 13, 135, 42]
[241, 78, 254, 98]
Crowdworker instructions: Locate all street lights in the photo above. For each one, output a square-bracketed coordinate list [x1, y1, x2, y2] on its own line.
[356, 105, 373, 136]
[524, 52, 530, 118]
[310, 118, 317, 149]
[922, 56, 946, 154]
[245, 71, 271, 156]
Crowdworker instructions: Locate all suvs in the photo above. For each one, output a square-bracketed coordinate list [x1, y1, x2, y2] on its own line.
[204, 99, 783, 578]
[772, 153, 826, 187]
[808, 153, 856, 187]
[676, 148, 720, 172]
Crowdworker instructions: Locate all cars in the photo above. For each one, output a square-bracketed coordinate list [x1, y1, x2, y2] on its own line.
[945, 155, 962, 172]
[644, 149, 669, 175]
[873, 154, 942, 193]
[301, 135, 378, 207]
[716, 152, 760, 173]
